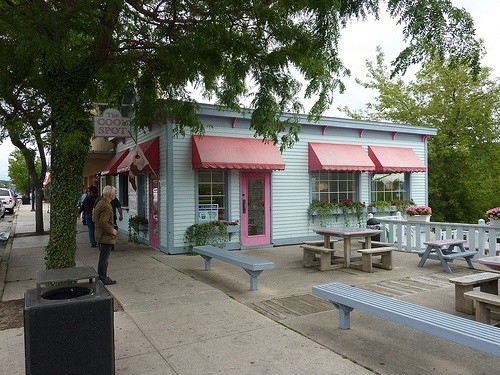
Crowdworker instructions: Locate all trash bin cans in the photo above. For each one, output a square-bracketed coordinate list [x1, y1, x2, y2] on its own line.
[24, 280, 115, 375]
[38, 265, 99, 287]
[367, 218, 380, 248]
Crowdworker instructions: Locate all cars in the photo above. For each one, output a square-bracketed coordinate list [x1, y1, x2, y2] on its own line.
[0, 198, 6, 219]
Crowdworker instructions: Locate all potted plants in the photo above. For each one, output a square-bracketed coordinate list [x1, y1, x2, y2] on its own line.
[128, 216, 146, 244]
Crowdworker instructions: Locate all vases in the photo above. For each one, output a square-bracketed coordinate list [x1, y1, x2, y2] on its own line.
[368, 205, 406, 213]
[407, 214, 430, 222]
[490, 217, 500, 225]
[192, 224, 241, 235]
[310, 206, 367, 214]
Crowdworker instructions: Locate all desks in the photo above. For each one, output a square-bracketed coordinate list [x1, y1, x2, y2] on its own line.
[478, 256, 500, 274]
[312, 228, 383, 268]
[418, 239, 475, 271]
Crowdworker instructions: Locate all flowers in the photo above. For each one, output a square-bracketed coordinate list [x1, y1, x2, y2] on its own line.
[311, 198, 364, 214]
[485, 207, 500, 219]
[206, 219, 239, 227]
[407, 206, 431, 216]
[371, 199, 412, 209]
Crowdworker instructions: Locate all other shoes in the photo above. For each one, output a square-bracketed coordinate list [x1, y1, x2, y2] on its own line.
[91, 245, 96, 248]
[83, 223, 87, 225]
[102, 280, 116, 285]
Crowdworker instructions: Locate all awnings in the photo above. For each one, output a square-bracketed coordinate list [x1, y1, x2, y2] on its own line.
[192, 134, 285, 171]
[117, 137, 160, 174]
[368, 145, 427, 174]
[308, 142, 375, 173]
[101, 148, 129, 176]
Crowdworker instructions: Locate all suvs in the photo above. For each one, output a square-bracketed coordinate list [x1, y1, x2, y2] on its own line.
[0, 187, 18, 214]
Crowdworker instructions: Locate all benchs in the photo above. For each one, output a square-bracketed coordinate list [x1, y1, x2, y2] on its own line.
[463, 290, 500, 323]
[450, 271, 500, 315]
[420, 246, 477, 260]
[313, 282, 500, 372]
[300, 239, 398, 272]
[193, 244, 273, 290]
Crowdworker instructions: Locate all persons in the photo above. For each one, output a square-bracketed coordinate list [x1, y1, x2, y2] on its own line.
[79, 186, 123, 251]
[93, 185, 117, 286]
